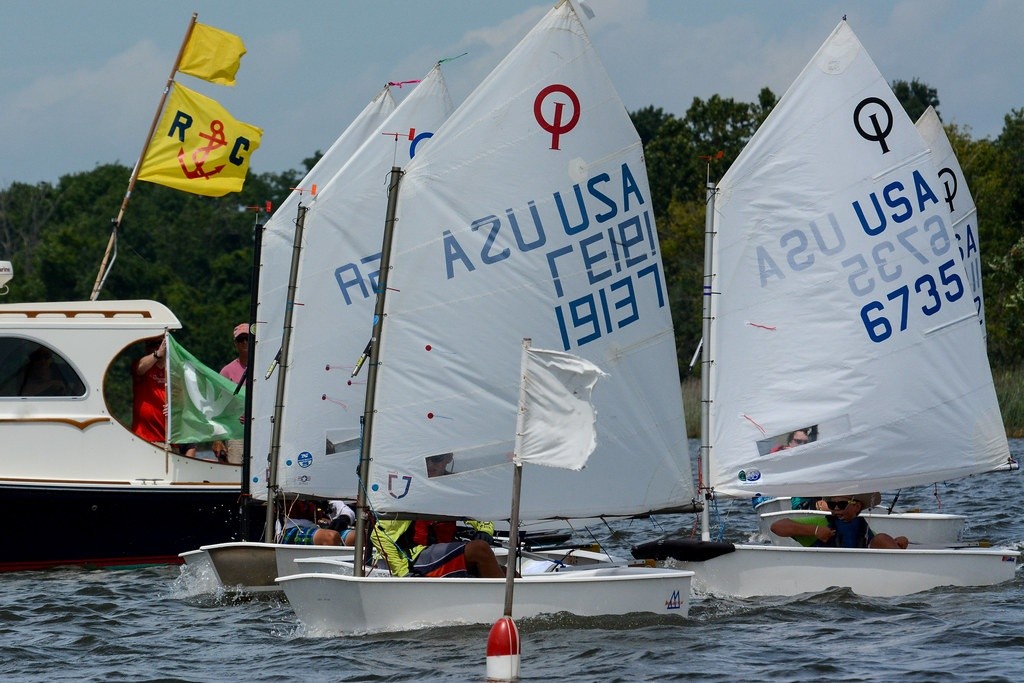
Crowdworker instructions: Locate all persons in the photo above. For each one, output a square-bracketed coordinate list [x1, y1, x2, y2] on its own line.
[211, 323, 249, 464]
[370, 452, 523, 578]
[770, 429, 812, 453]
[130, 335, 179, 453]
[791, 496, 822, 511]
[274, 439, 364, 546]
[770, 495, 909, 550]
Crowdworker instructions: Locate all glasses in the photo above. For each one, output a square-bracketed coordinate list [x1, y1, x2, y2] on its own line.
[426, 455, 446, 462]
[792, 439, 808, 444]
[827, 500, 855, 510]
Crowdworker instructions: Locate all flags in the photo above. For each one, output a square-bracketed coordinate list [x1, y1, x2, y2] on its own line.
[512, 346, 612, 472]
[177, 20, 248, 87]
[137, 80, 264, 197]
[165, 332, 246, 444]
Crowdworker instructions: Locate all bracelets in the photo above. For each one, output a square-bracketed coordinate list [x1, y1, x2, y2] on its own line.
[153, 350, 162, 360]
[815, 525, 819, 536]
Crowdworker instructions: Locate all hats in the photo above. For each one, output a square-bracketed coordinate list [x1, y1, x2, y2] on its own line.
[234, 323, 249, 339]
[823, 492, 881, 509]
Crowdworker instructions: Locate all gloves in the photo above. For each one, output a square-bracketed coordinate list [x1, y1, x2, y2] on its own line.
[328, 515, 350, 531]
[473, 532, 493, 545]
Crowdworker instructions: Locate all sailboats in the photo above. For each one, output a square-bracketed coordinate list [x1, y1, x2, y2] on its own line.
[176, 0, 1024, 635]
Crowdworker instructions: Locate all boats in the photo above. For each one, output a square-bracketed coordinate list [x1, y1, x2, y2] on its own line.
[0, 11, 362, 557]
